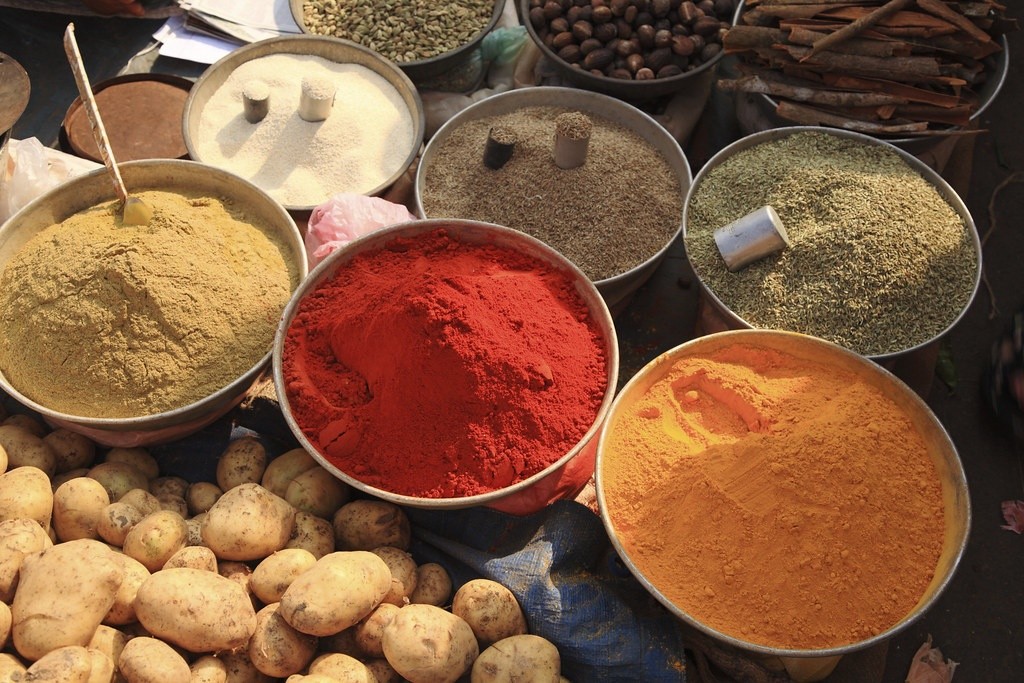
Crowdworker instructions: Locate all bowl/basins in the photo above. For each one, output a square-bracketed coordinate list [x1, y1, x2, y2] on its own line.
[520, 0, 726, 96]
[731, 0, 1011, 143]
[415, 85, 691, 293]
[289, 0, 506, 68]
[680, 123, 983, 357]
[181, 34, 424, 215]
[64, 73, 194, 164]
[0, 155, 309, 445]
[595, 329, 971, 656]
[271, 218, 618, 510]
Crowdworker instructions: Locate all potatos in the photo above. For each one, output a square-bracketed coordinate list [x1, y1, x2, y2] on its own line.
[0, 406, 568, 683]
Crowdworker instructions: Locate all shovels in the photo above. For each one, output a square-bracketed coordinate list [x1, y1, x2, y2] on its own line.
[64, 22, 153, 225]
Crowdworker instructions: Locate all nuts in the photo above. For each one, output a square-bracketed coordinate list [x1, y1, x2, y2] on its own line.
[301, 0, 496, 63]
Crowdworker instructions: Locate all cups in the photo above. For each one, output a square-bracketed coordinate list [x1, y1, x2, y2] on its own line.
[711, 206, 786, 272]
[483, 125, 517, 170]
[242, 87, 271, 123]
[298, 83, 337, 122]
[554, 128, 590, 169]
